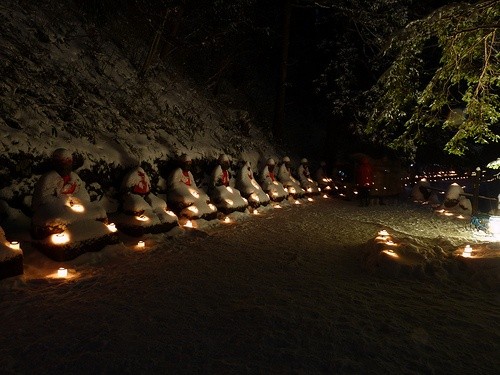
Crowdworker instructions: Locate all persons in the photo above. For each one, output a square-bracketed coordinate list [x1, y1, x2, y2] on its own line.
[278, 156, 300, 186]
[357, 158, 372, 207]
[166, 163, 210, 206]
[297, 158, 318, 188]
[123, 161, 167, 216]
[32, 148, 108, 234]
[261, 158, 283, 191]
[212, 155, 240, 200]
[236, 161, 262, 195]
[316, 162, 331, 185]
